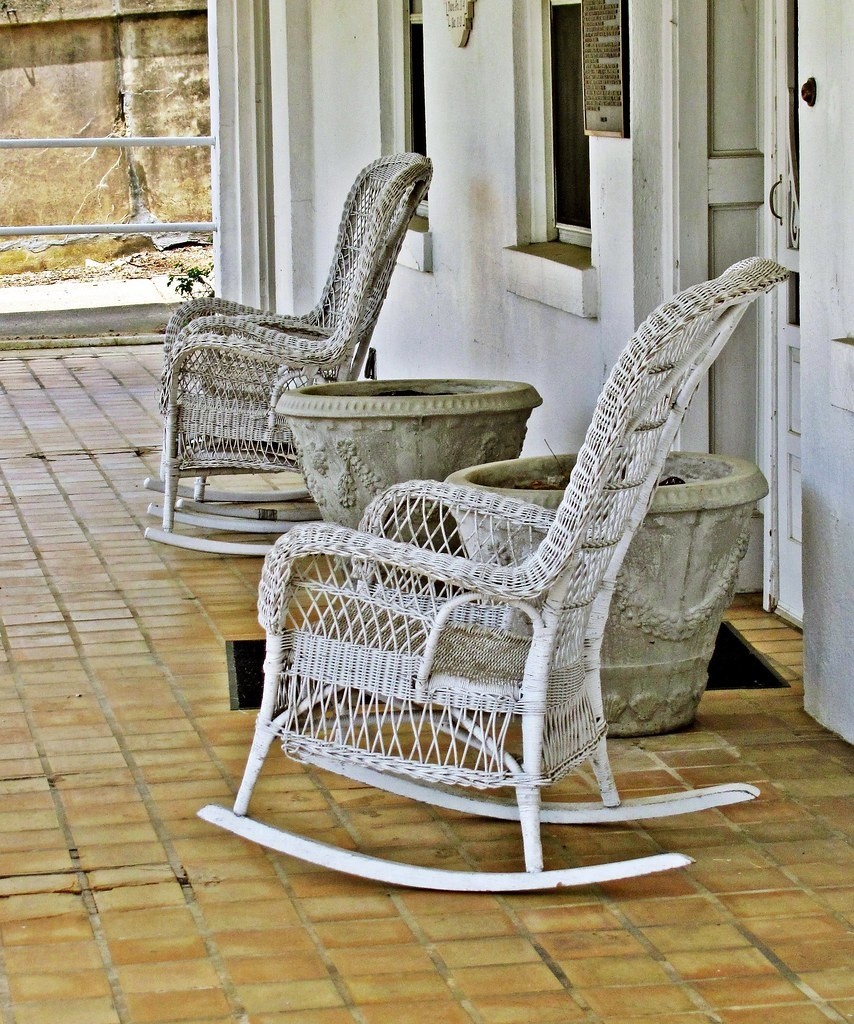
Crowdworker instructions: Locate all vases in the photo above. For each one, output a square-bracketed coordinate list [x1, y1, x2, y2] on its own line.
[275, 378, 544, 530]
[442, 451, 769, 738]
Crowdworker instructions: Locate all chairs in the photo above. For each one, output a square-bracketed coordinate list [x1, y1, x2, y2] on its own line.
[144, 153, 433, 556]
[196, 254, 795, 892]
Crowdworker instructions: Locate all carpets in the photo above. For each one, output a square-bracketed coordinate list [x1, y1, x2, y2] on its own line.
[225, 620, 790, 710]
